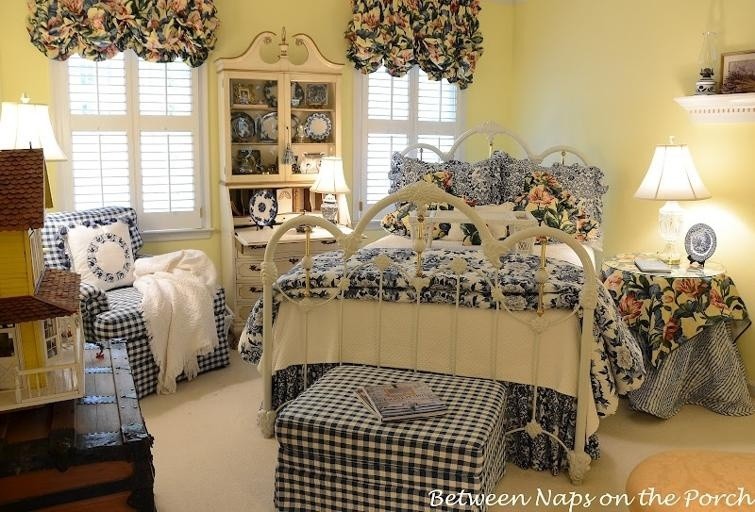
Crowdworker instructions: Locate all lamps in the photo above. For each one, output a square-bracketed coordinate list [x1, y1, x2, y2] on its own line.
[0, 93, 69, 161]
[309, 157, 353, 228]
[632, 136, 713, 265]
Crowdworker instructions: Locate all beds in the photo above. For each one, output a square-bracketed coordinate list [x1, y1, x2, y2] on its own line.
[256, 120, 604, 489]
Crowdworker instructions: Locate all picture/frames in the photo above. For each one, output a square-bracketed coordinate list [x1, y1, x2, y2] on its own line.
[718, 49, 755, 92]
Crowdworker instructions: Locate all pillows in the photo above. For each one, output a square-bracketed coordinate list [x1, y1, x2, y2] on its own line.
[462, 172, 601, 248]
[389, 150, 502, 209]
[380, 171, 477, 242]
[60, 215, 139, 295]
[491, 151, 610, 240]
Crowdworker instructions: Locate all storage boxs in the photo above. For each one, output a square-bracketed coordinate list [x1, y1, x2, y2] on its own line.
[0, 337, 159, 512]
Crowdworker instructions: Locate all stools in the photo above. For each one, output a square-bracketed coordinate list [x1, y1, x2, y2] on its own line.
[275, 363, 508, 512]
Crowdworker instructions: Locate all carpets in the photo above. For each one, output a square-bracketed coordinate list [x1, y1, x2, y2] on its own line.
[624, 448, 755, 511]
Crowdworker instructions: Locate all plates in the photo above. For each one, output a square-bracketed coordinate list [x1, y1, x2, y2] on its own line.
[259, 111, 303, 143]
[232, 112, 257, 142]
[305, 113, 333, 143]
[682, 224, 716, 263]
[249, 191, 279, 228]
[262, 80, 304, 108]
[306, 85, 329, 106]
[236, 148, 263, 176]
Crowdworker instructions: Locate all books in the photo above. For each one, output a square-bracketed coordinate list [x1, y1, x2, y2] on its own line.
[634, 258, 671, 272]
[354, 382, 447, 421]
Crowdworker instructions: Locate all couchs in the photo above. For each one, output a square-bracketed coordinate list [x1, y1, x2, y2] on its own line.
[38, 210, 233, 402]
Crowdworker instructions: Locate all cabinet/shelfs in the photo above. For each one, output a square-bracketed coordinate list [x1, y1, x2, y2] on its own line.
[213, 26, 355, 350]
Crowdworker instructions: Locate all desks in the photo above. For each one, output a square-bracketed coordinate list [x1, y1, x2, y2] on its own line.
[599, 255, 729, 420]
